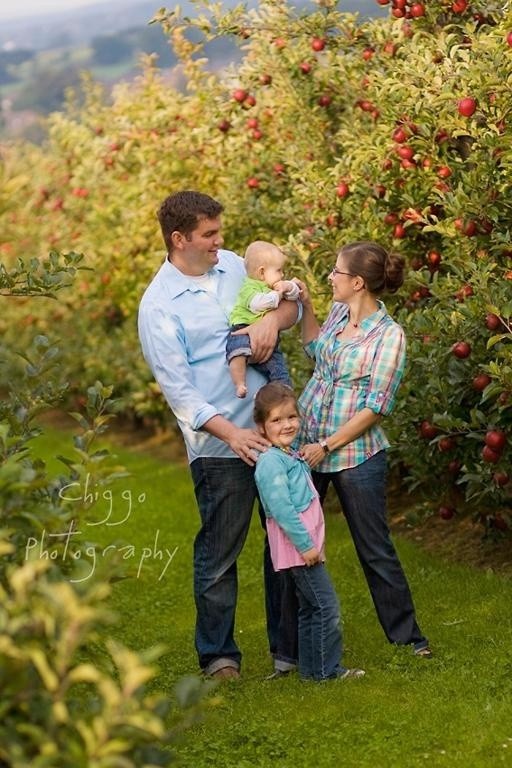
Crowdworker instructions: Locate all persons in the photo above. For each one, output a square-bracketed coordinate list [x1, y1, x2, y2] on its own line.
[138, 191, 304, 681]
[263, 242, 433, 679]
[252, 380, 365, 682]
[225, 241, 300, 398]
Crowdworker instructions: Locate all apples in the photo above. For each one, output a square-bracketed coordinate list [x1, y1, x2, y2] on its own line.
[0, 0, 512, 524]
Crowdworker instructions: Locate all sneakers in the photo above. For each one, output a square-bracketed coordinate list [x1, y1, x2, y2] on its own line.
[213, 668, 366, 686]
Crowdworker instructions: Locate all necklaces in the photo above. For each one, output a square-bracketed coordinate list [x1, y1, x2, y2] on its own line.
[354, 323, 357, 328]
[272, 444, 305, 463]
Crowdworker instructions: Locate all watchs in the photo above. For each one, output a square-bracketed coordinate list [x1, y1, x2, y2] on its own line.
[320, 441, 330, 455]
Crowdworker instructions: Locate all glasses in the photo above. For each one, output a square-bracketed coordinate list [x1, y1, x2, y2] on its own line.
[332, 268, 355, 277]
